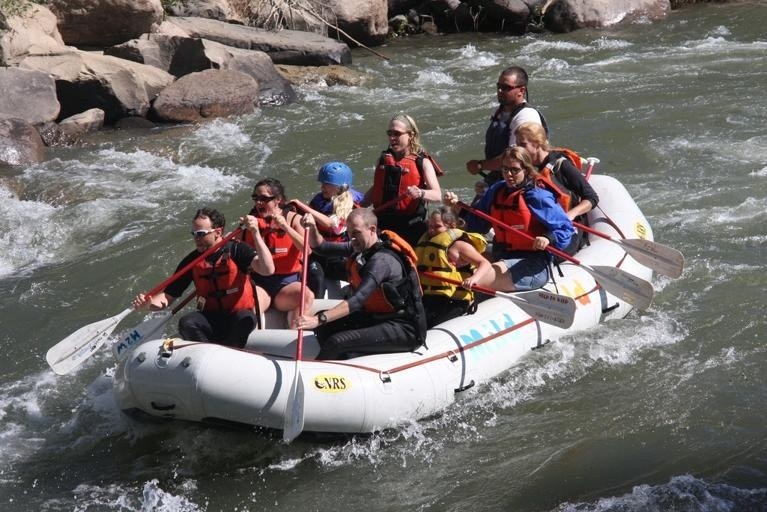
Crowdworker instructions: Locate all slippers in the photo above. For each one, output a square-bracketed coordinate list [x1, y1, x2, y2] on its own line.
[251, 193, 280, 203]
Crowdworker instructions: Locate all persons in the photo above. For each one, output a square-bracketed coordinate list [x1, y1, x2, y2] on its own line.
[473, 122, 600, 266]
[232, 177, 315, 331]
[465, 66, 551, 205]
[357, 112, 443, 250]
[412, 203, 491, 330]
[131, 207, 277, 350]
[443, 145, 574, 292]
[291, 208, 427, 360]
[287, 162, 366, 298]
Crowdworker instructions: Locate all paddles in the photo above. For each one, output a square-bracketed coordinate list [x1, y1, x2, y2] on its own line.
[111, 288, 196, 360]
[571, 221, 684, 279]
[419, 270, 575, 329]
[283, 225, 310, 446]
[46, 222, 247, 376]
[457, 201, 653, 311]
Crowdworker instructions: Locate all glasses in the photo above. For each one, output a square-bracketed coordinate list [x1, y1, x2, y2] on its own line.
[384, 128, 412, 136]
[495, 80, 528, 92]
[499, 165, 528, 174]
[188, 227, 223, 238]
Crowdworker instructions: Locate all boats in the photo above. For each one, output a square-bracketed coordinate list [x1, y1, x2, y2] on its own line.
[111, 173, 656, 434]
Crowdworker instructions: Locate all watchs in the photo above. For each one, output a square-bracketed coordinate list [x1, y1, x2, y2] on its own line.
[318, 310, 328, 324]
[478, 159, 484, 171]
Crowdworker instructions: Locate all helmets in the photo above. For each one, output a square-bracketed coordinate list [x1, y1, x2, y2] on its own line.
[316, 160, 354, 190]
